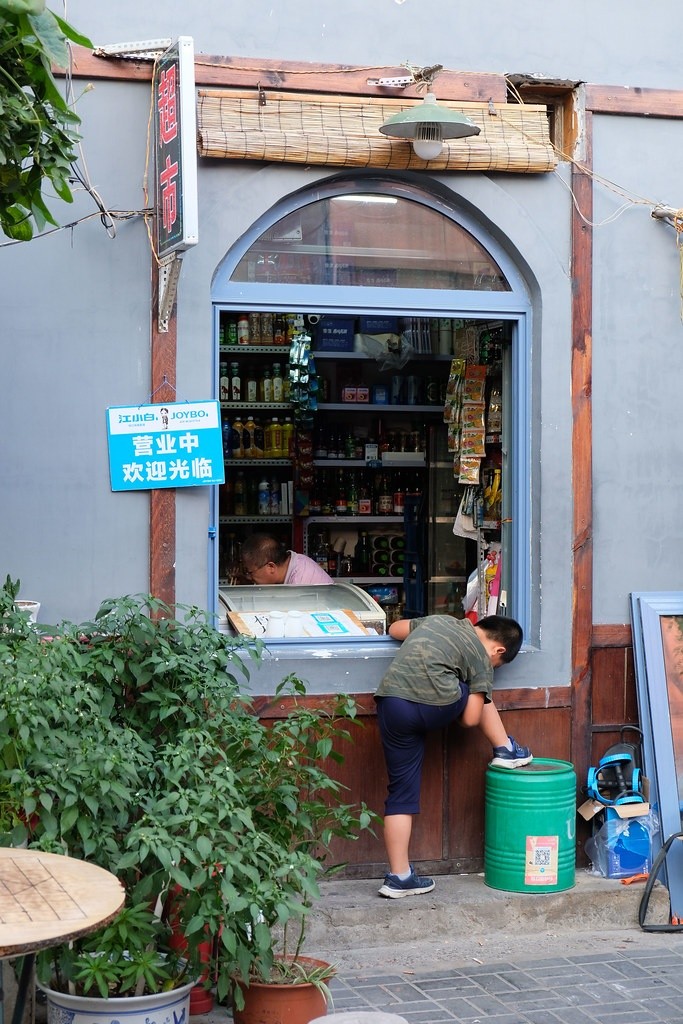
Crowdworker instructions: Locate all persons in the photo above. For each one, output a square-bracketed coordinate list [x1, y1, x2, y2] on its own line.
[373, 614, 532, 898]
[240, 533, 333, 585]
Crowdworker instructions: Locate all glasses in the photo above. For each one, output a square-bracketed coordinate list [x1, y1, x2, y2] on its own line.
[243, 563, 268, 576]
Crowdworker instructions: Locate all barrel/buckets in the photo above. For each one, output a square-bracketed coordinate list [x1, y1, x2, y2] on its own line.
[483, 758, 577, 893]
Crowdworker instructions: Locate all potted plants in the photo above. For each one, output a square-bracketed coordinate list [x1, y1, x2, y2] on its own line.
[0, 576, 384, 1024]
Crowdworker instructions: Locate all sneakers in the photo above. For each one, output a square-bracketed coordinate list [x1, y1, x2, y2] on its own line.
[378, 870, 434, 899]
[491, 736, 533, 769]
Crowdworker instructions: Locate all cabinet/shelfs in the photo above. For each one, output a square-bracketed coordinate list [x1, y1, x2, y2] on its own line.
[221, 308, 503, 641]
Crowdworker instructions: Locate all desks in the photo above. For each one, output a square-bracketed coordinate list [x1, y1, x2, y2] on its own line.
[0, 845, 126, 1024]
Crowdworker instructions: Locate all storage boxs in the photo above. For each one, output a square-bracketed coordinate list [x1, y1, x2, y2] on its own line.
[356, 387, 369, 402]
[358, 315, 398, 335]
[342, 387, 357, 402]
[314, 313, 358, 352]
[370, 384, 388, 405]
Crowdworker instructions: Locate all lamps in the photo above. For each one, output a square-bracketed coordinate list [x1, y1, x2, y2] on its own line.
[378, 90, 483, 162]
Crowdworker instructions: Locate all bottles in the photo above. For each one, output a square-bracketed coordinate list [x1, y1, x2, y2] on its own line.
[217, 307, 510, 587]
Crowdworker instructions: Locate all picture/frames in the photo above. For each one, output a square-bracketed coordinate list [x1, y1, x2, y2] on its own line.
[627, 590, 683, 929]
